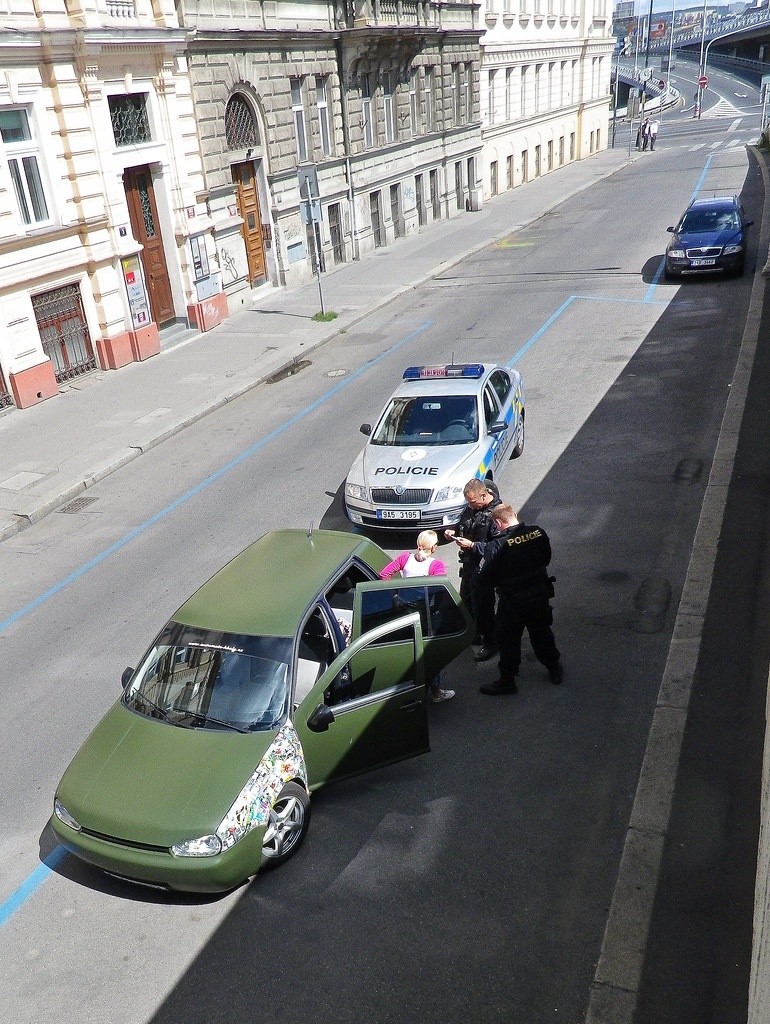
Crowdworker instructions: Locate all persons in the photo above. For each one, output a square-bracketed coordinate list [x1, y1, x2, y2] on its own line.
[378, 530, 446, 581]
[473, 501, 564, 695]
[444, 479, 503, 663]
[642, 118, 650, 152]
[650, 119, 658, 151]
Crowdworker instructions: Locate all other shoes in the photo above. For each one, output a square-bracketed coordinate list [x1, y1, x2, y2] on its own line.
[473, 647, 497, 662]
[651, 149, 655, 151]
[432, 689, 455, 703]
[644, 149, 646, 152]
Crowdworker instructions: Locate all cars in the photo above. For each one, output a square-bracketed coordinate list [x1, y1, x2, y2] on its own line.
[50, 523, 476, 894]
[341, 362, 527, 532]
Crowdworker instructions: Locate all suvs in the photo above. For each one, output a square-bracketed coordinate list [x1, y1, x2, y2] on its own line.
[665, 195, 754, 280]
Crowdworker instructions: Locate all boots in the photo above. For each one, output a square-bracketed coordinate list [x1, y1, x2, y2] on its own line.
[545, 661, 563, 685]
[479, 670, 518, 696]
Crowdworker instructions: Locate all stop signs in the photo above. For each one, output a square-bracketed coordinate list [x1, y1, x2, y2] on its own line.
[699, 76, 708, 88]
[658, 80, 665, 90]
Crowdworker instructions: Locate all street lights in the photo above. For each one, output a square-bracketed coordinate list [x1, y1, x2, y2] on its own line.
[615, 43, 631, 108]
